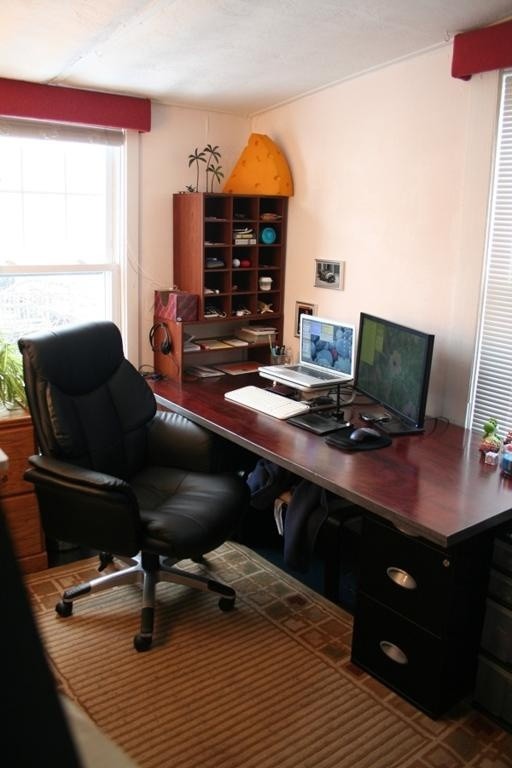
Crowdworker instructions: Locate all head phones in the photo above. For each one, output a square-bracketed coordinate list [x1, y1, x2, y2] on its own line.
[139, 365, 162, 382]
[149, 322, 171, 355]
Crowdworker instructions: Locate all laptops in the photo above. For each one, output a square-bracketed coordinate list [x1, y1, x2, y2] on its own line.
[257, 313, 355, 387]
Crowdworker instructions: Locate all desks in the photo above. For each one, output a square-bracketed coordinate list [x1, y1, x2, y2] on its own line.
[142, 355, 511, 723]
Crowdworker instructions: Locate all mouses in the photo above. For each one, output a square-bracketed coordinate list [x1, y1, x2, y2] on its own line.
[349, 427, 382, 442]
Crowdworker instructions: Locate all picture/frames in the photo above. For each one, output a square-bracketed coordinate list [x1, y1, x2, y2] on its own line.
[314, 258, 344, 291]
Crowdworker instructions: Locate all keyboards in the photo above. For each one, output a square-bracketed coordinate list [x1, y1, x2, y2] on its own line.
[223, 385, 310, 420]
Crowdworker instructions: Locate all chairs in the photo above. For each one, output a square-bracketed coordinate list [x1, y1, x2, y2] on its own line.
[6, 330, 254, 655]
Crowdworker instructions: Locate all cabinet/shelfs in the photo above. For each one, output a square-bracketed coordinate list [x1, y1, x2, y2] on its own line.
[153, 193, 289, 385]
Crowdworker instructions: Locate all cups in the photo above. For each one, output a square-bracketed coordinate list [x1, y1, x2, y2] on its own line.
[500, 442, 512, 479]
[270, 354, 290, 367]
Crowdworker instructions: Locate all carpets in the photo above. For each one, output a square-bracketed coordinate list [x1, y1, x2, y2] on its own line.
[20, 545, 511, 765]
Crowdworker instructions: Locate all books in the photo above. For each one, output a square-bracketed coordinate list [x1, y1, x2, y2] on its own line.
[182, 326, 279, 353]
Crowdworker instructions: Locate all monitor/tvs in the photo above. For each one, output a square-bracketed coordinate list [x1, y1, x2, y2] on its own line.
[353, 312, 435, 435]
